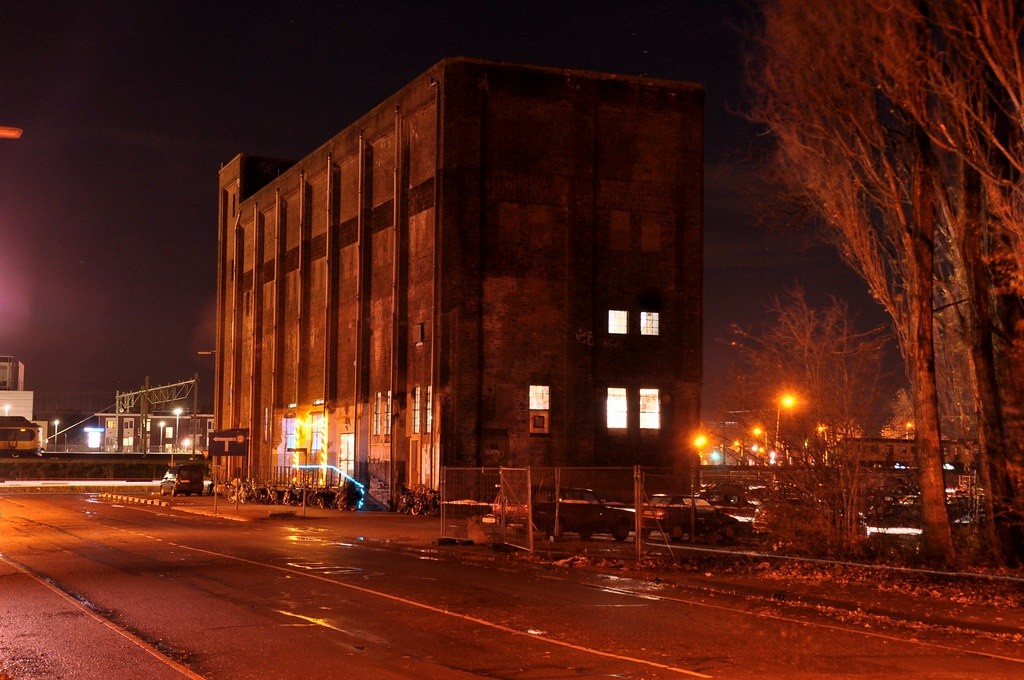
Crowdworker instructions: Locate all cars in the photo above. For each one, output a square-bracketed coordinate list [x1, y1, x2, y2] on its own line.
[872, 490, 964, 532]
[641, 494, 743, 543]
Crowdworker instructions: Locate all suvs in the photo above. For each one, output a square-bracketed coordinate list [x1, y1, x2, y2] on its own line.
[492, 484, 634, 542]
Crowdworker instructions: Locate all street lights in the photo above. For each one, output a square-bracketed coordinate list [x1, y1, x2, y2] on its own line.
[775, 391, 799, 460]
[53, 419, 59, 452]
[158, 421, 166, 453]
[174, 408, 183, 452]
[818, 424, 828, 460]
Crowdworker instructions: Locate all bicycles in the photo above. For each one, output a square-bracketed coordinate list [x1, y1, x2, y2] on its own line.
[396, 481, 441, 516]
[225, 476, 360, 512]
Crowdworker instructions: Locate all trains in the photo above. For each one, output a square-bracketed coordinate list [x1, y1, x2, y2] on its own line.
[0, 416, 43, 458]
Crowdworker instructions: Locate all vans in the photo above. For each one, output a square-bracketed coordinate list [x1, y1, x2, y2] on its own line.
[159, 464, 205, 497]
[753, 497, 869, 535]
